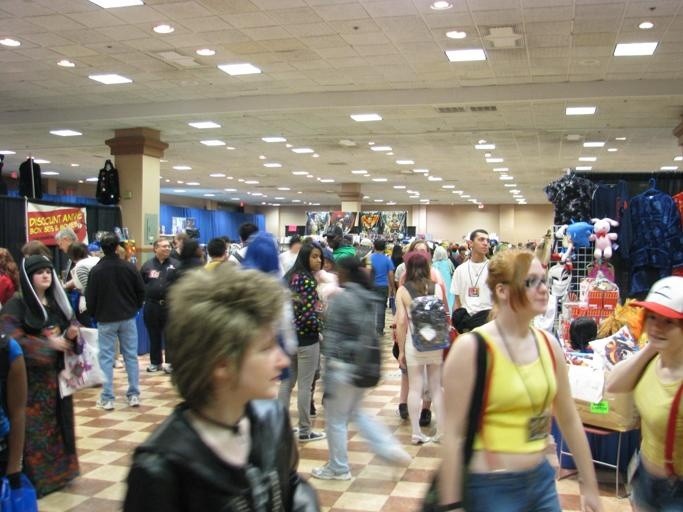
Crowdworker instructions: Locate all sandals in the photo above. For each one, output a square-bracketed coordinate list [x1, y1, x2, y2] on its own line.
[411, 433, 431, 445]
[433, 434, 443, 442]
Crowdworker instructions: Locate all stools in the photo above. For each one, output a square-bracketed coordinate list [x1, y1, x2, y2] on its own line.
[556, 425, 641, 498]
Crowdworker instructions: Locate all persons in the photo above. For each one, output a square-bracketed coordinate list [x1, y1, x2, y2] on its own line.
[0, 218, 683, 512]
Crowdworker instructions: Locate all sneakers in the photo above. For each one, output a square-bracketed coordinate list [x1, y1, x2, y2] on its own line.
[128, 395, 139, 407]
[396, 403, 408, 419]
[307, 399, 317, 417]
[419, 408, 432, 426]
[311, 464, 353, 481]
[295, 431, 326, 443]
[144, 362, 161, 372]
[94, 398, 116, 411]
[162, 362, 174, 373]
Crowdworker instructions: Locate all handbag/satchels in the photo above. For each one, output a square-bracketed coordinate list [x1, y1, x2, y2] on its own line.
[338, 325, 382, 388]
[54, 333, 106, 399]
[421, 467, 469, 512]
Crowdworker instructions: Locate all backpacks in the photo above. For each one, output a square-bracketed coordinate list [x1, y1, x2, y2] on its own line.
[403, 281, 452, 353]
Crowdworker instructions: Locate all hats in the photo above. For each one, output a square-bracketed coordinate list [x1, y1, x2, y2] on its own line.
[322, 224, 343, 238]
[24, 253, 54, 274]
[628, 275, 683, 323]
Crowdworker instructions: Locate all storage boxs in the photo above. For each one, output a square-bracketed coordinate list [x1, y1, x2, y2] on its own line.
[568, 372, 643, 433]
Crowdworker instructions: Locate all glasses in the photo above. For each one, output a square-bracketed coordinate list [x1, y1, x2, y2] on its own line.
[507, 273, 547, 289]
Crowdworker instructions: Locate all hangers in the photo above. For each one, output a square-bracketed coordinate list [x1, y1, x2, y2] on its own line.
[642, 178, 660, 196]
